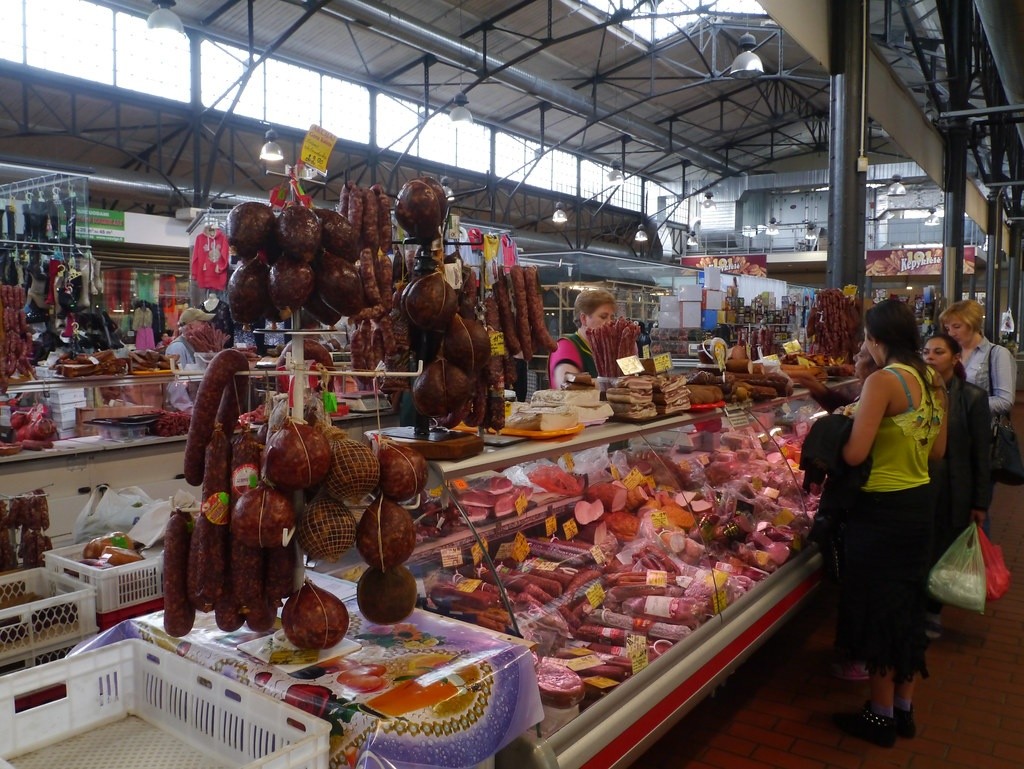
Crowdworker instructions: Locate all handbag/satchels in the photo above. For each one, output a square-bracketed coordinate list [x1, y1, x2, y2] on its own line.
[992, 421, 1024, 486]
[926, 522, 1011, 615]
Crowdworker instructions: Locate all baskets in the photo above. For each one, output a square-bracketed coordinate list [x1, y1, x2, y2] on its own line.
[0, 566, 100, 678]
[40, 533, 165, 614]
[0, 637, 333, 769]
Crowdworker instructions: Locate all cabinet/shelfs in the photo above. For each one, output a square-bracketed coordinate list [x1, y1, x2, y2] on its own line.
[368, 372, 867, 768]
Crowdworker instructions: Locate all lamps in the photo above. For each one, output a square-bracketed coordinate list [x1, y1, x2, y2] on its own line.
[608, 22, 626, 183]
[762, 121, 817, 240]
[887, 144, 906, 197]
[925, 180, 940, 226]
[551, 141, 568, 223]
[702, 167, 716, 210]
[634, 169, 649, 241]
[260, 48, 284, 161]
[147, 0, 183, 37]
[687, 164, 700, 247]
[450, 0, 474, 125]
[729, 0, 765, 78]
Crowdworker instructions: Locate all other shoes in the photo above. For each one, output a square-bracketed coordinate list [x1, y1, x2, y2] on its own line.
[833, 660, 870, 680]
[831, 705, 896, 747]
[867, 700, 915, 738]
[926, 612, 943, 640]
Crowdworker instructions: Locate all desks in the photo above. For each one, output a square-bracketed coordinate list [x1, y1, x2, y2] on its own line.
[64, 567, 546, 769]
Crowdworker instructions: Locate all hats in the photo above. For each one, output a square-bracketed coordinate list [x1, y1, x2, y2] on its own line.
[179, 308, 216, 325]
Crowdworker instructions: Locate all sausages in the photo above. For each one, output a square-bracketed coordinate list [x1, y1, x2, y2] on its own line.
[0, 175, 578, 710]
[578, 286, 923, 703]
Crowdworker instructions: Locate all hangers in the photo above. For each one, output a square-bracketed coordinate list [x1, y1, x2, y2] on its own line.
[203, 222, 218, 236]
[470, 223, 511, 241]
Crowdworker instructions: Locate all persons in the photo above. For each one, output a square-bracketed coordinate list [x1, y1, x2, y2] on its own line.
[825, 298, 948, 748]
[832, 338, 877, 680]
[547, 291, 638, 390]
[938, 298, 1017, 444]
[637, 321, 651, 358]
[920, 333, 995, 642]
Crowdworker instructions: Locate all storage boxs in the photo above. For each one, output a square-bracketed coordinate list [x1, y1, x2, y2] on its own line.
[0, 637, 333, 768]
[0, 535, 167, 676]
